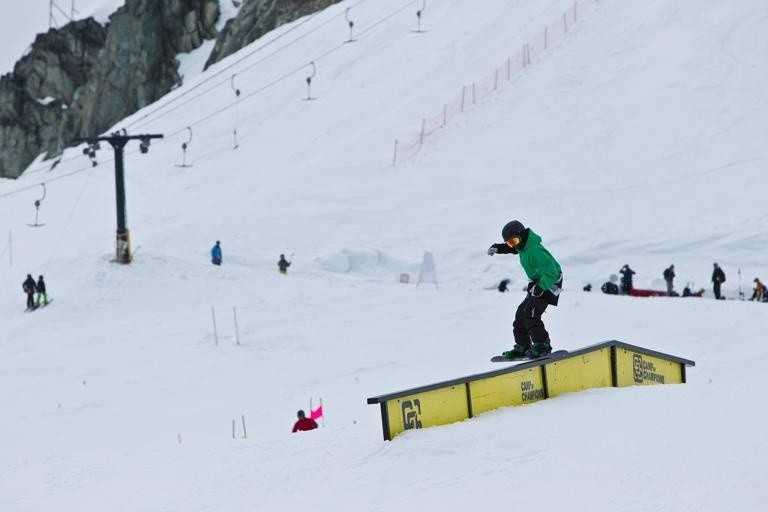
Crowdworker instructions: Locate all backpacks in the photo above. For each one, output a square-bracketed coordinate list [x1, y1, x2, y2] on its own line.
[663, 269, 669, 280]
[719, 268, 726, 282]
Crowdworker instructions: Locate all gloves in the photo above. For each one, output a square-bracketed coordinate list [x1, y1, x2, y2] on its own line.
[530, 285, 543, 298]
[488, 246, 498, 257]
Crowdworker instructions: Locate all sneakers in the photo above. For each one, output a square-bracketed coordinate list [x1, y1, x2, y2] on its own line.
[505, 343, 552, 358]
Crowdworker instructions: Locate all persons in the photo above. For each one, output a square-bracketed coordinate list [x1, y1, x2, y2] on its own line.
[211, 240, 223, 265]
[749, 277, 768, 303]
[619, 264, 636, 295]
[681, 282, 705, 297]
[278, 254, 291, 275]
[498, 278, 510, 292]
[664, 265, 675, 295]
[35, 275, 48, 307]
[292, 410, 318, 432]
[583, 284, 592, 291]
[712, 263, 725, 299]
[23, 274, 38, 309]
[488, 221, 563, 360]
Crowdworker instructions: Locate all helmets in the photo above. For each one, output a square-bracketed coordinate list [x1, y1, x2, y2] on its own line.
[502, 220, 525, 242]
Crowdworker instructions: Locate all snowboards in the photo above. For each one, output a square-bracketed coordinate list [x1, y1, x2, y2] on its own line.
[491, 350, 568, 362]
